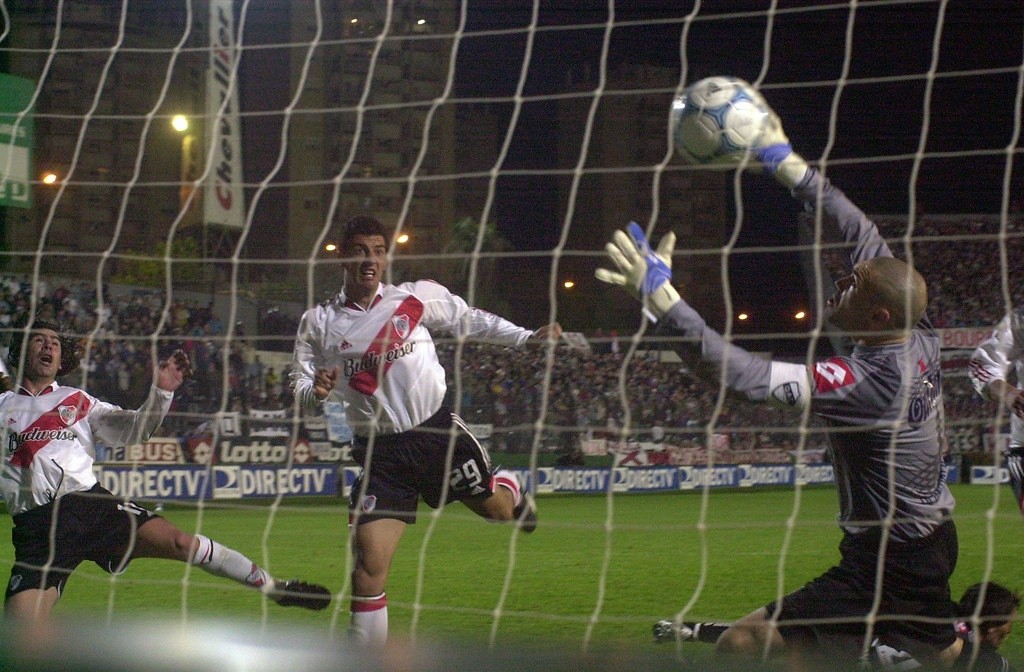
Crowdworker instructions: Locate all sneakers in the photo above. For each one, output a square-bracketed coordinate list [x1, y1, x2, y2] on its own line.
[266, 579, 331, 610]
[653, 620, 702, 643]
[500, 468, 537, 532]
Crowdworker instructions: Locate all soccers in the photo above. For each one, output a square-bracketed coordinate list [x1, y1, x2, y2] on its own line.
[670, 74, 768, 169]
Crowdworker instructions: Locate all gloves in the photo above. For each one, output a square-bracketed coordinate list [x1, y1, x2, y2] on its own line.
[750, 113, 806, 189]
[594, 220, 680, 325]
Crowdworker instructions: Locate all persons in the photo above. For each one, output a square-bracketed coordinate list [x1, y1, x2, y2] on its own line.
[0, 321, 332, 653]
[969, 303, 1023, 514]
[595, 115, 1014, 672]
[290, 217, 562, 661]
[654, 581, 1023, 672]
[0, 214, 1024, 464]
